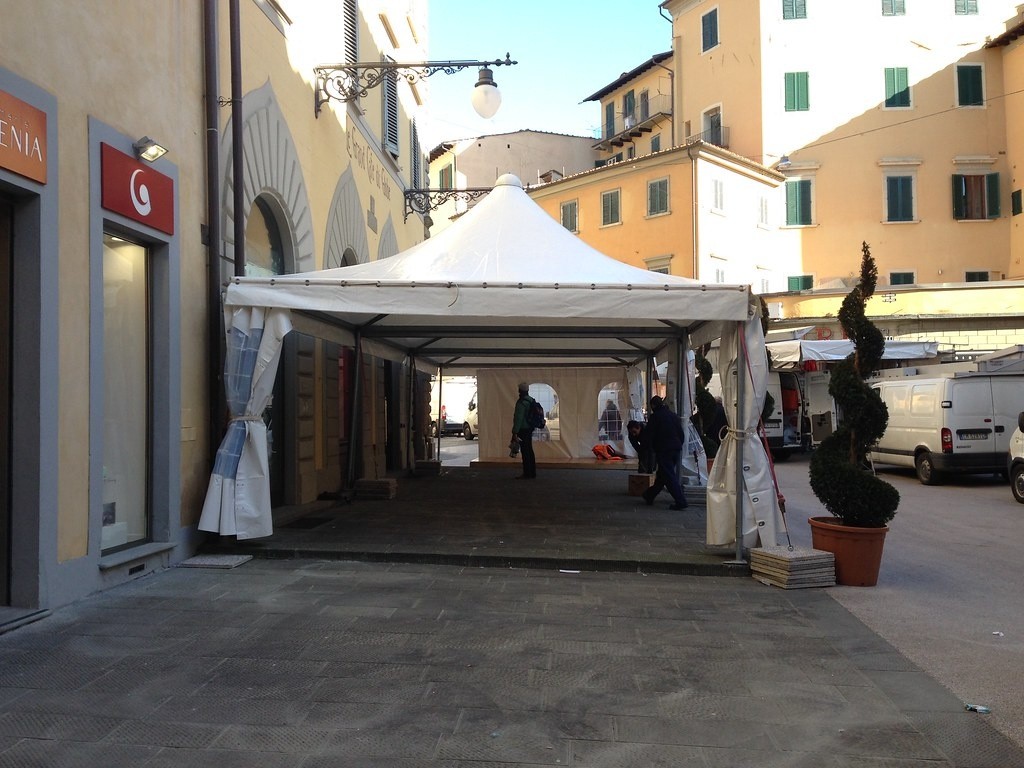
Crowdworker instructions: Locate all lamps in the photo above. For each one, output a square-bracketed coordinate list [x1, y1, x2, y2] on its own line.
[133, 136, 168, 161]
[314, 53, 518, 119]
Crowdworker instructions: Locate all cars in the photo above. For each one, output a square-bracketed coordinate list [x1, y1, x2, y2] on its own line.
[463, 372, 651, 441]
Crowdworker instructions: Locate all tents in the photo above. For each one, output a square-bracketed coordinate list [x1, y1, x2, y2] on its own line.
[198, 170, 779, 570]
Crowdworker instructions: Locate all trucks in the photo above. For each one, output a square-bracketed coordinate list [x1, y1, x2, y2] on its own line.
[861, 364, 1024, 488]
[662, 326, 837, 464]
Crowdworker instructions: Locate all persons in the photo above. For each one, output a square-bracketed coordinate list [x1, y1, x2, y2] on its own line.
[512, 383, 537, 479]
[599, 399, 622, 440]
[641, 395, 690, 509]
[627, 421, 657, 474]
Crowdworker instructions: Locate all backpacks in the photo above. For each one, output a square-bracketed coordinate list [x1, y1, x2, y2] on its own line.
[521, 398, 546, 429]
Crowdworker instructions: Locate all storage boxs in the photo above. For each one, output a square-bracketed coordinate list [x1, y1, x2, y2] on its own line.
[629, 473, 655, 496]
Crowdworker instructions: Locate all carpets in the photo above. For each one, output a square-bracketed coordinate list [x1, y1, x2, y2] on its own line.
[282, 517, 333, 530]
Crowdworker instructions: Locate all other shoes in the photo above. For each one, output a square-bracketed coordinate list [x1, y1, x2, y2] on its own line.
[669, 504, 688, 510]
[514, 473, 536, 480]
[641, 491, 652, 505]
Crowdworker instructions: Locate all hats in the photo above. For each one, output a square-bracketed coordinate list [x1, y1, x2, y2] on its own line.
[518, 381, 529, 391]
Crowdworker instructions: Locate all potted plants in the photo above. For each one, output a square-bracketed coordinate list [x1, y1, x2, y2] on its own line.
[808, 240, 900, 588]
[689, 339, 719, 474]
